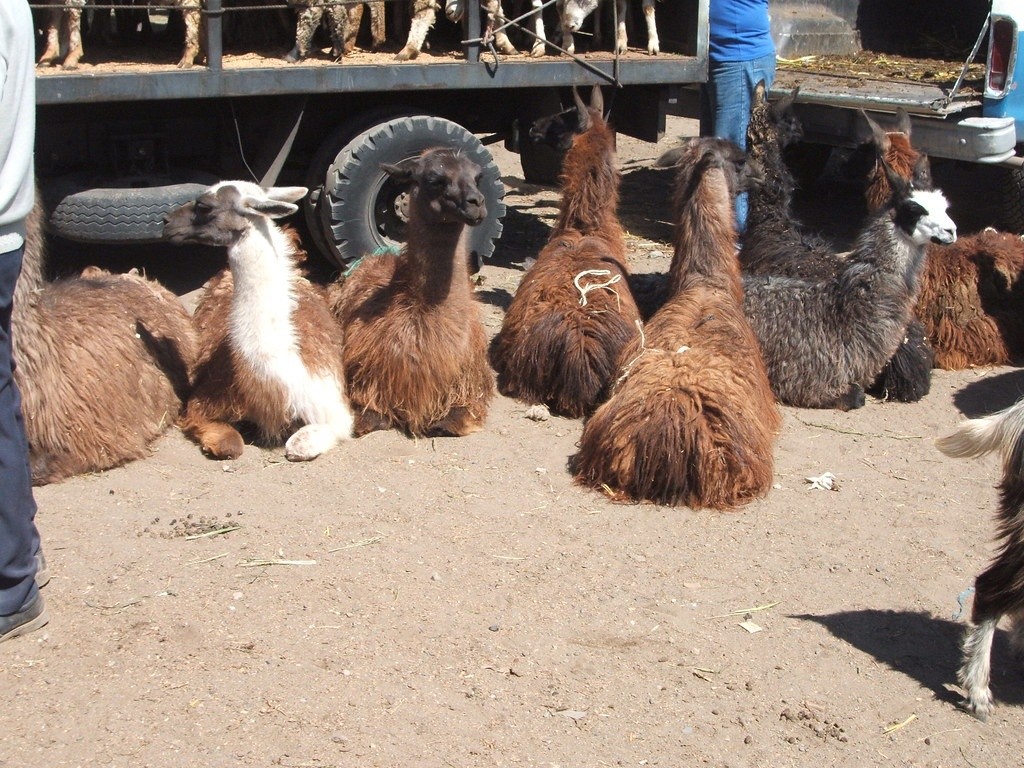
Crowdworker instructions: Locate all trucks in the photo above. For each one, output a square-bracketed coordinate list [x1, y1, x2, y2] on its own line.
[25, 0, 708, 294]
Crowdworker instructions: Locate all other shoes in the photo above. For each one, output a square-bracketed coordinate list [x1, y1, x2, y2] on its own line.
[0, 549, 52, 645]
[734, 235, 744, 252]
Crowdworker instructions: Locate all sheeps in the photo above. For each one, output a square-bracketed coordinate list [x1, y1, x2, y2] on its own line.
[12, 145, 492, 487]
[934, 400, 1024, 720]
[489, 78, 779, 509]
[737, 79, 1024, 408]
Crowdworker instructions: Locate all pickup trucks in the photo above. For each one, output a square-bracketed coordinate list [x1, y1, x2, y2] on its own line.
[669, 0, 1024, 168]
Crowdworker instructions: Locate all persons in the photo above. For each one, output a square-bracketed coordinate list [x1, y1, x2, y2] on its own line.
[708, 0, 780, 238]
[0, 0, 52, 644]
